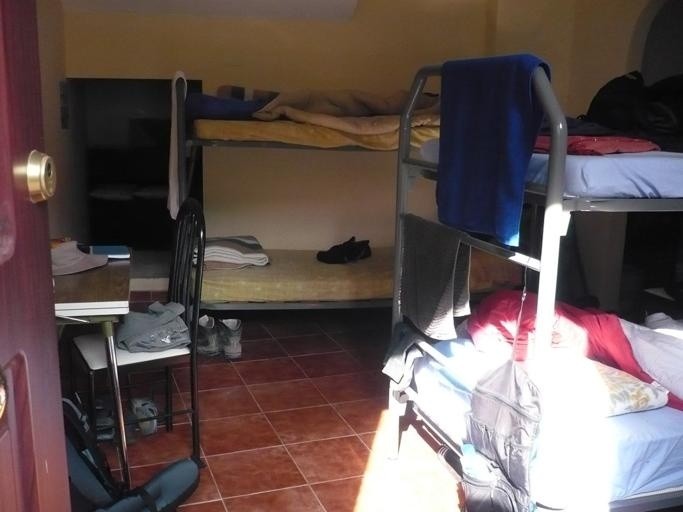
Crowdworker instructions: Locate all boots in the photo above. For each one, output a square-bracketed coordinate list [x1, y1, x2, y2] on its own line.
[220, 319, 241, 360]
[198, 315, 219, 356]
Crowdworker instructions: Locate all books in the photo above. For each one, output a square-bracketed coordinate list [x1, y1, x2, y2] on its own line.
[90, 245, 131, 259]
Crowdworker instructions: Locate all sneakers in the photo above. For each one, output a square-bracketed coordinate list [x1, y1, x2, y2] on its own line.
[94, 406, 114, 439]
[133, 398, 158, 435]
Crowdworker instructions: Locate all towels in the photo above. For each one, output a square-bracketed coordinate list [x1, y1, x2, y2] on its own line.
[164, 72, 190, 220]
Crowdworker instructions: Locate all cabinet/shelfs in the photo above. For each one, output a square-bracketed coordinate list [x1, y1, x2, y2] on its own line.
[65, 77, 202, 280]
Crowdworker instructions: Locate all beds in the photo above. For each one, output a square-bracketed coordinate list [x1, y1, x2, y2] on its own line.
[172, 55, 682, 510]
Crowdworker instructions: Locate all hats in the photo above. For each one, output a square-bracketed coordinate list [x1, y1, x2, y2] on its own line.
[51, 240, 109, 275]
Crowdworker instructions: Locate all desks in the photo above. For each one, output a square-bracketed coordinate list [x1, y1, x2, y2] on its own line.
[50, 247, 133, 493]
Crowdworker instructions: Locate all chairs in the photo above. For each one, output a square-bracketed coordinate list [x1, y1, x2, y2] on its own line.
[67, 197, 206, 490]
